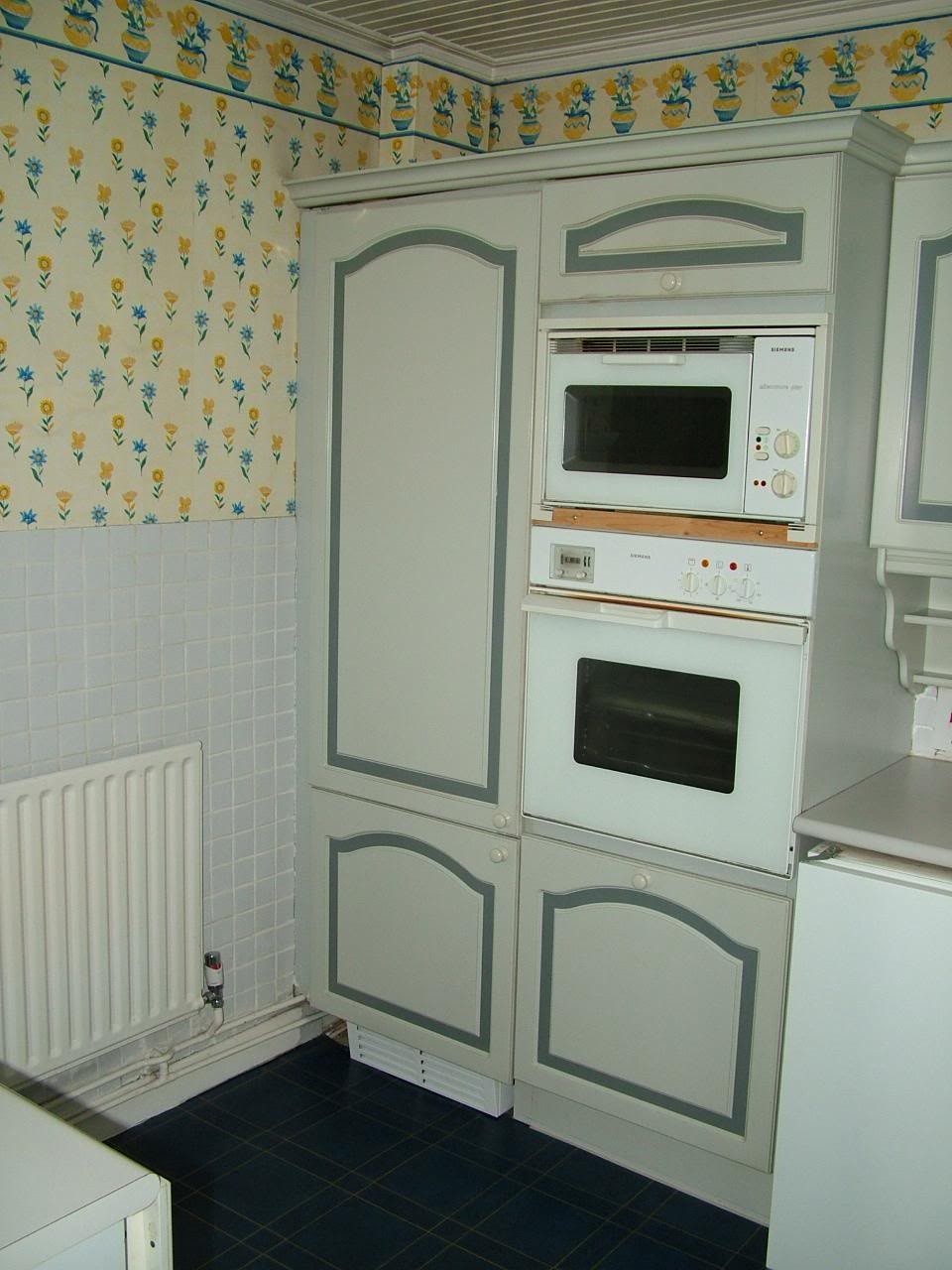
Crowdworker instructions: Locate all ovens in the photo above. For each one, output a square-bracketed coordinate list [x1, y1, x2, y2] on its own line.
[522, 525, 817, 879]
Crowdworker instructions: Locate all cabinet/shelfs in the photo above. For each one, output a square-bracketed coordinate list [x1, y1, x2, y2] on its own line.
[300, 119, 952, 1234]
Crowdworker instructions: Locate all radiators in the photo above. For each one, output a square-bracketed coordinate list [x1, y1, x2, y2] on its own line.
[4, 746, 226, 1090]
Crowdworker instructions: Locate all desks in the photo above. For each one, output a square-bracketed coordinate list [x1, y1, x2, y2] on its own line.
[0, 1090, 174, 1270]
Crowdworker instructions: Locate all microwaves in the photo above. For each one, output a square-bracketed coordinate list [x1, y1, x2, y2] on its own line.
[540, 328, 817, 523]
